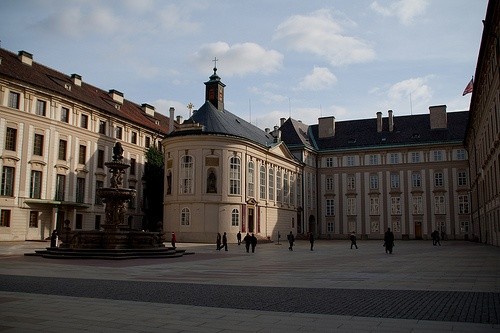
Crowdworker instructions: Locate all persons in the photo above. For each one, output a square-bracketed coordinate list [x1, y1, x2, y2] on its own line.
[219, 232, 228, 251]
[431, 229, 440, 246]
[244, 232, 251, 253]
[383, 227, 395, 254]
[237, 231, 241, 245]
[350, 231, 359, 249]
[287, 231, 295, 251]
[171, 231, 176, 247]
[216, 232, 221, 250]
[309, 232, 315, 251]
[250, 233, 257, 253]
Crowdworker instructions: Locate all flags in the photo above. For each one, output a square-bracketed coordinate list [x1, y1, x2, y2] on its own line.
[462, 78, 475, 96]
